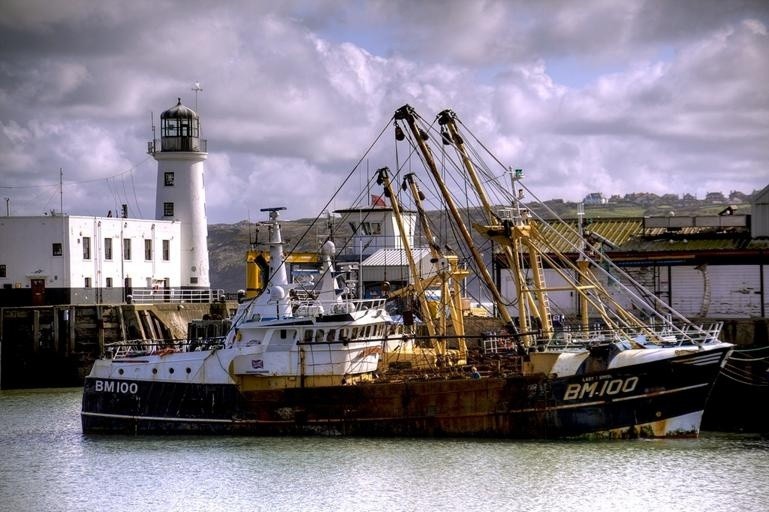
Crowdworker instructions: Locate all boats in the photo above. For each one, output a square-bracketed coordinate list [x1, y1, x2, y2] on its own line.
[77, 102, 739, 443]
